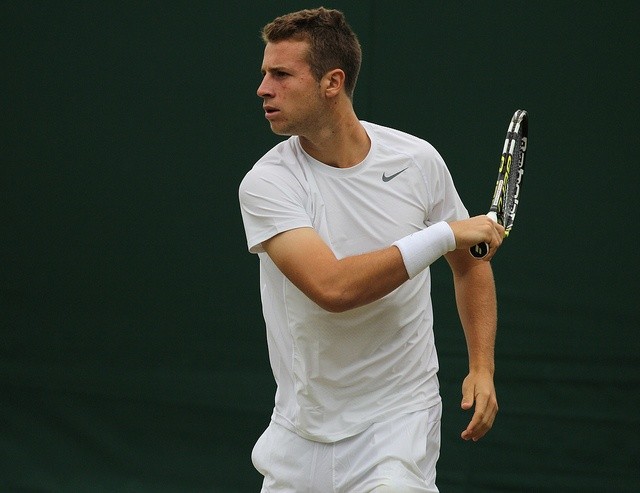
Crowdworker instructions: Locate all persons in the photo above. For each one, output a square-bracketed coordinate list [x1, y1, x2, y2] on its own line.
[237, 5, 507, 493]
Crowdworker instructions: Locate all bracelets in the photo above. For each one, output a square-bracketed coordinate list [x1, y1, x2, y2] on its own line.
[391, 221, 456, 279]
